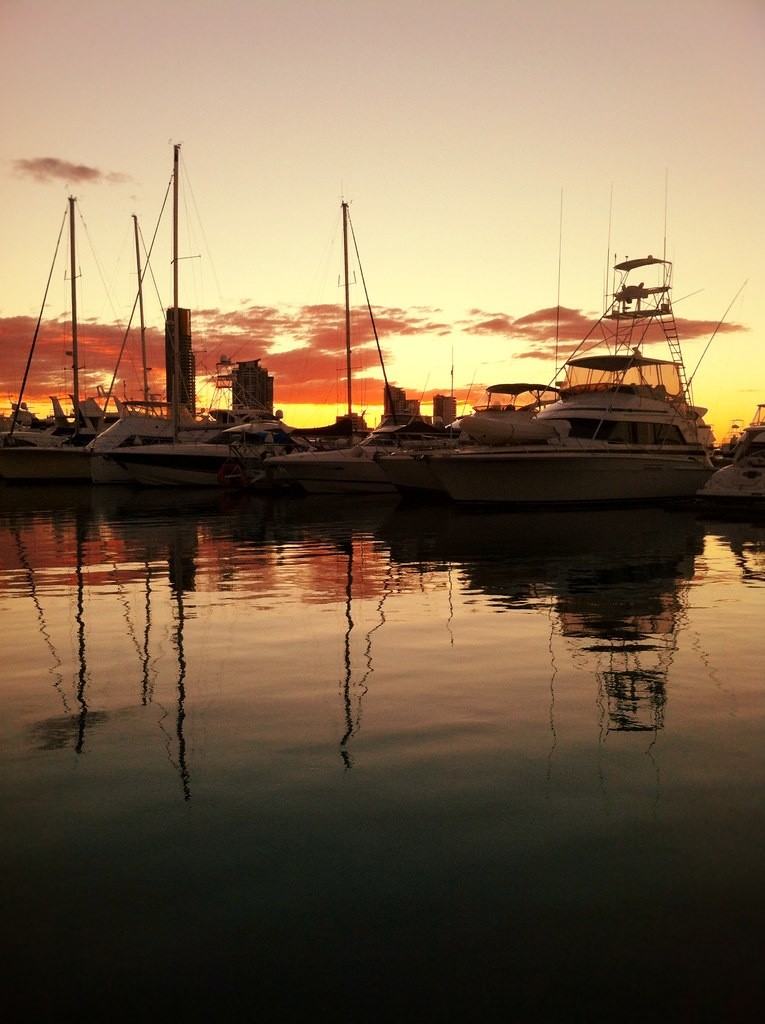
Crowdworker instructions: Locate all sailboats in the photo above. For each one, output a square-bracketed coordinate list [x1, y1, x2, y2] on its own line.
[0, 141, 764, 508]
